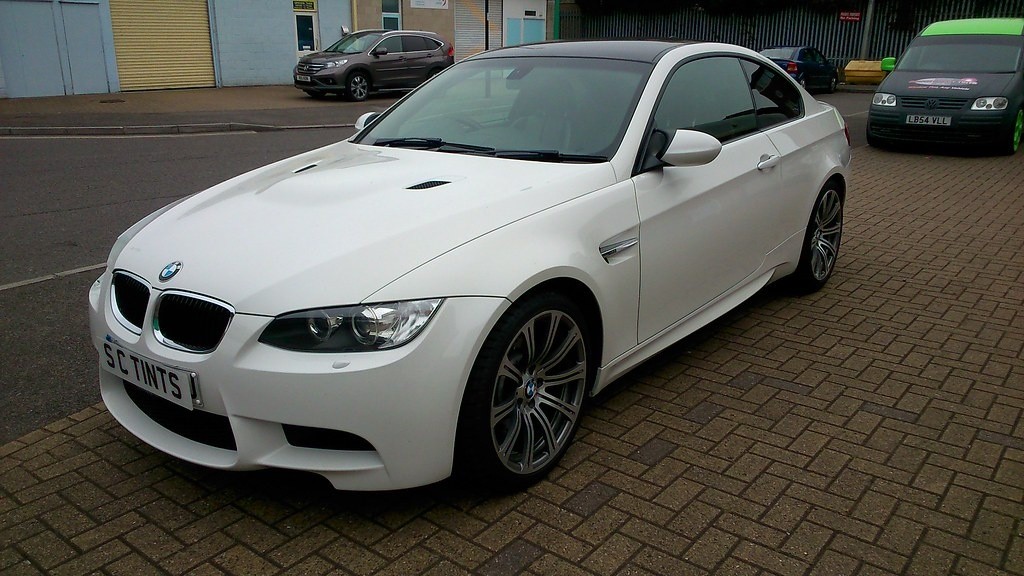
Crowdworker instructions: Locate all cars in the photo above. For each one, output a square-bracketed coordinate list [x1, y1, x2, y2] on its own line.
[867, 16, 1024, 154]
[88, 34, 854, 488]
[758, 46, 839, 95]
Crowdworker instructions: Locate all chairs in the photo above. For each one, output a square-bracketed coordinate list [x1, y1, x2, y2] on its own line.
[507, 72, 577, 124]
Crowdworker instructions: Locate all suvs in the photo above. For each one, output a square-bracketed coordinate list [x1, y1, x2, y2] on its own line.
[293, 27, 456, 100]
